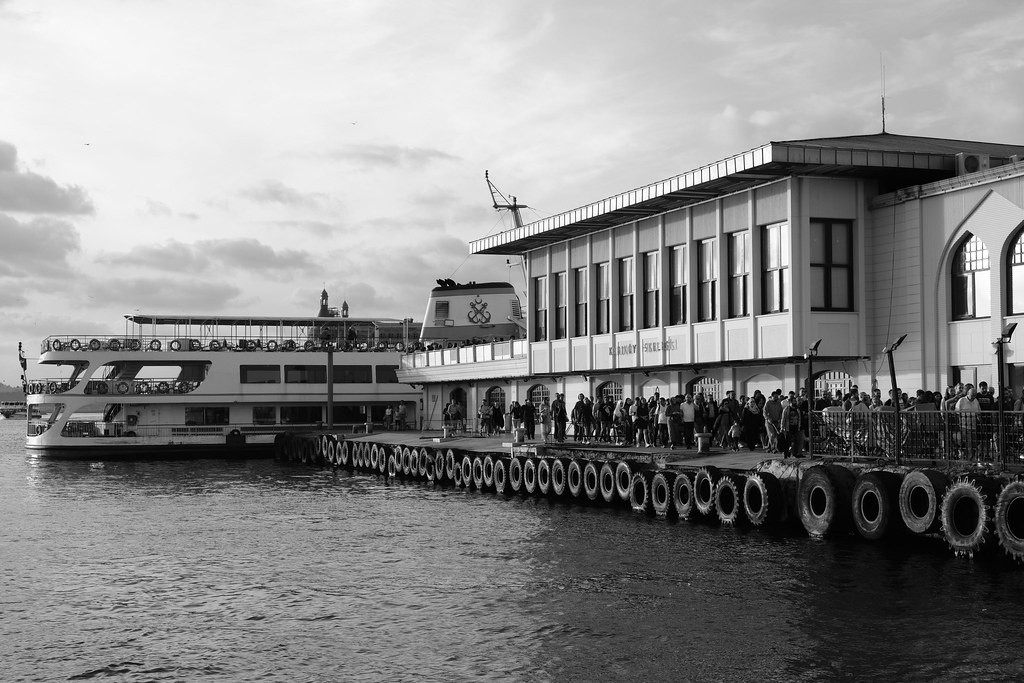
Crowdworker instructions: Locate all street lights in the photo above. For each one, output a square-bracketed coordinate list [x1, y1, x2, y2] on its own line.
[807, 338, 822, 459]
[996, 322, 1018, 464]
[883, 333, 908, 465]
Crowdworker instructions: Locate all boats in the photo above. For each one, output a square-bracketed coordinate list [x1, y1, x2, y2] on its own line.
[18, 313, 424, 462]
[0, 400, 27, 420]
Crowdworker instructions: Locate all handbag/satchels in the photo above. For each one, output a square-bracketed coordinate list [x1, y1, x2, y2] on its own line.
[777, 430, 787, 450]
[540, 412, 548, 423]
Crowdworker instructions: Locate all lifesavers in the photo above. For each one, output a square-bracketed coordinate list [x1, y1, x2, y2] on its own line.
[28, 382, 58, 394]
[96, 381, 190, 394]
[285, 340, 296, 351]
[109, 339, 121, 351]
[274, 430, 1024, 564]
[90, 338, 101, 350]
[231, 429, 241, 436]
[150, 339, 161, 351]
[35, 425, 43, 435]
[209, 340, 221, 350]
[190, 339, 201, 350]
[245, 340, 256, 351]
[267, 340, 278, 352]
[304, 340, 405, 352]
[127, 415, 137, 425]
[70, 339, 81, 350]
[52, 339, 62, 351]
[129, 339, 142, 351]
[170, 339, 182, 351]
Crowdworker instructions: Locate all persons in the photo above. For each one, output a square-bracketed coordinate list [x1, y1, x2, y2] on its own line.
[384, 382, 1023, 457]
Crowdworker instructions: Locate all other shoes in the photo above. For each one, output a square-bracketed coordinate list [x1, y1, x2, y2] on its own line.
[796, 454, 806, 458]
[784, 449, 788, 458]
[450, 430, 698, 452]
[714, 440, 777, 453]
